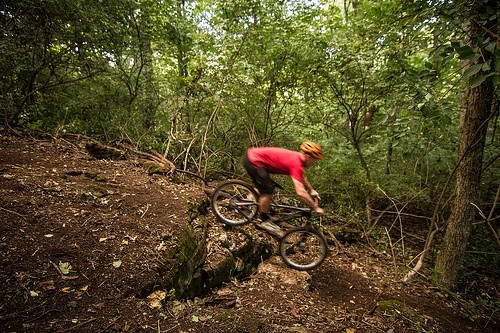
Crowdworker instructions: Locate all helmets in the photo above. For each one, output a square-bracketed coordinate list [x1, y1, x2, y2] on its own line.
[300, 141, 323, 160]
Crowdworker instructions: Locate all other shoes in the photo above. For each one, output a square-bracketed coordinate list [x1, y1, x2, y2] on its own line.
[261, 220, 284, 237]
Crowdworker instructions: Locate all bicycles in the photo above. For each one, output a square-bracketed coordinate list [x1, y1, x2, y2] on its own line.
[211, 177, 329, 271]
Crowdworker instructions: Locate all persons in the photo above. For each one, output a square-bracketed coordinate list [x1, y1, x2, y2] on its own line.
[243, 140, 325, 233]
